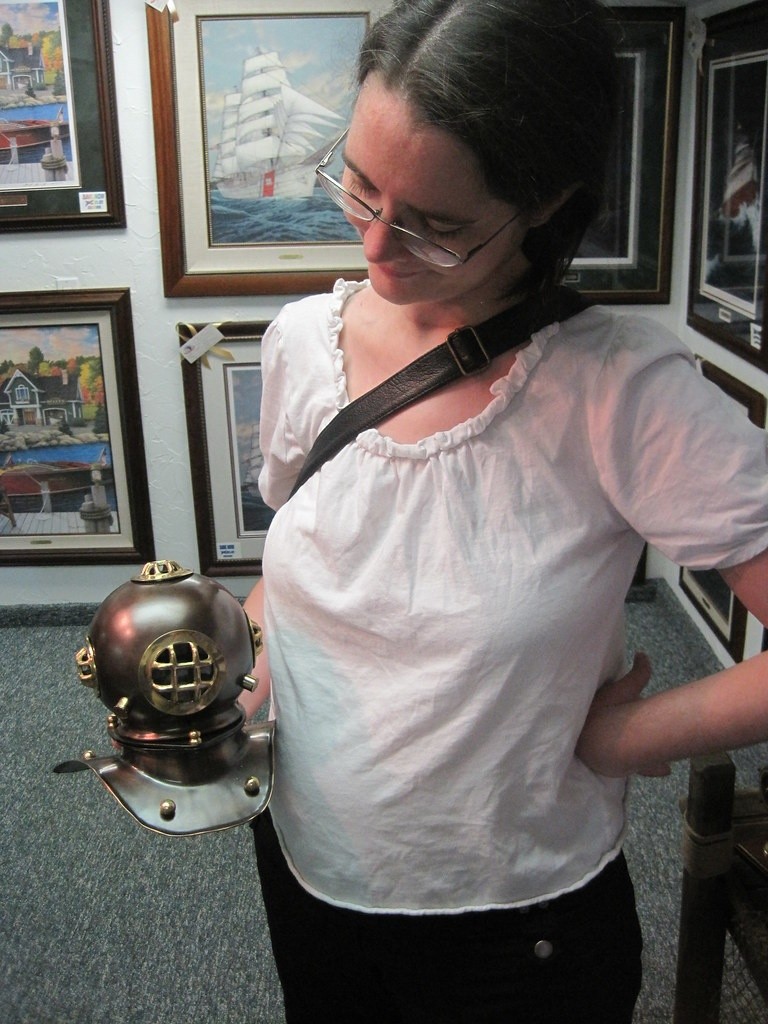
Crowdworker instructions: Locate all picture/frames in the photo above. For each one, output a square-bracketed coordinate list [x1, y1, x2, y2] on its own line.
[0, 0, 127, 238]
[686, 0, 768, 374]
[1, 287, 157, 566]
[145, 1, 398, 299]
[177, 321, 277, 578]
[555, 5, 687, 305]
[678, 357, 768, 664]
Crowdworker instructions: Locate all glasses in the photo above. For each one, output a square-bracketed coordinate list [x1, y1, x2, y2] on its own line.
[313, 126, 527, 268]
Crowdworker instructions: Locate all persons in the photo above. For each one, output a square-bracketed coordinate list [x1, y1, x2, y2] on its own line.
[109, 0, 768, 1024]
